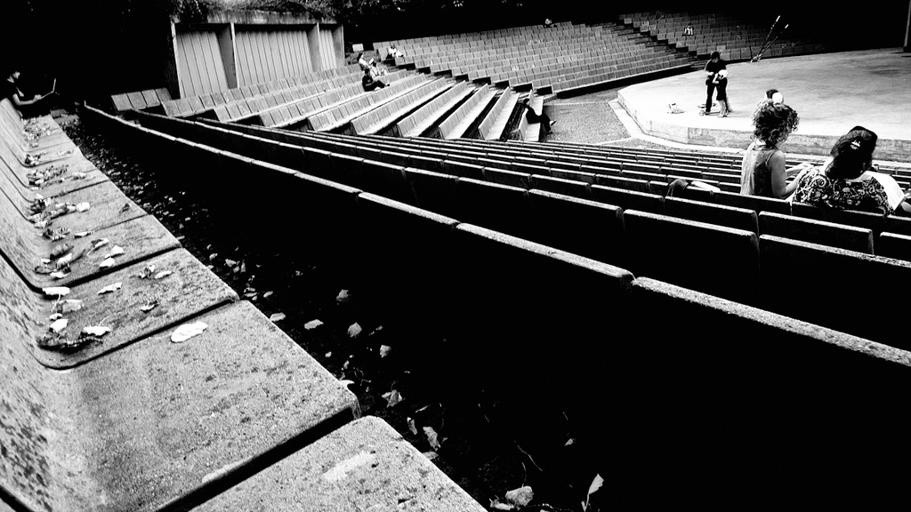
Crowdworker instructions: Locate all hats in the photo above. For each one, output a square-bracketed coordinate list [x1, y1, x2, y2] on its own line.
[830, 125, 877, 171]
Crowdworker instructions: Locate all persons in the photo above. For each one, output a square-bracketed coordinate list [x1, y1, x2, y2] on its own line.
[681, 23, 693, 35]
[739, 87, 816, 200]
[712, 70, 728, 118]
[785, 137, 896, 216]
[703, 51, 732, 115]
[372, 61, 391, 85]
[4, 65, 73, 118]
[387, 44, 405, 67]
[817, 126, 911, 212]
[522, 98, 557, 135]
[544, 17, 558, 28]
[362, 67, 390, 92]
[357, 53, 373, 72]
[640, 8, 673, 21]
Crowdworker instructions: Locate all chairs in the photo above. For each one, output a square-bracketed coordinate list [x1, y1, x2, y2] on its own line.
[2, 99, 911, 510]
[110, 1, 794, 98]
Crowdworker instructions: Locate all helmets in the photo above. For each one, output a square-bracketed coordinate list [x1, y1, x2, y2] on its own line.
[719, 70, 728, 78]
